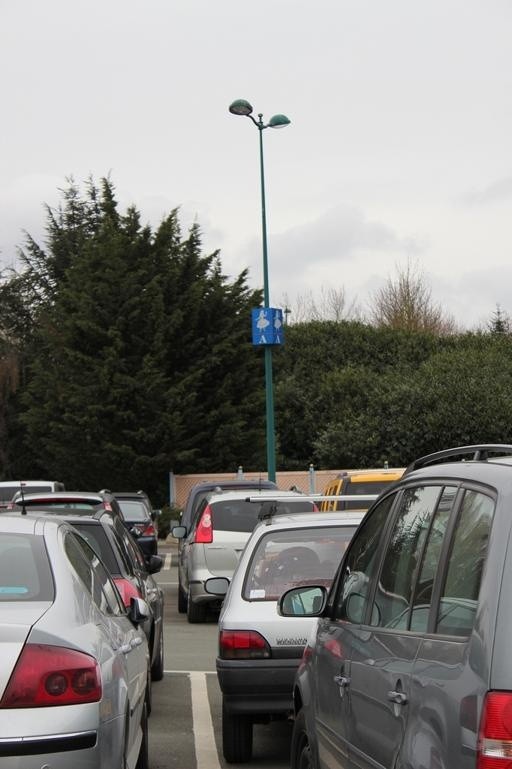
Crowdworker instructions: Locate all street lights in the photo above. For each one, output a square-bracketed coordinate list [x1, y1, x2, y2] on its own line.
[228, 98, 292, 483]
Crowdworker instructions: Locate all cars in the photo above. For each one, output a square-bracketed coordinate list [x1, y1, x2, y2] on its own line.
[214, 494, 379, 763]
[2, 509, 153, 769]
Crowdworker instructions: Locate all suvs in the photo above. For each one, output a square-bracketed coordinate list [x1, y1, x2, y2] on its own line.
[275, 442, 511, 769]
[171, 485, 320, 624]
[175, 477, 279, 549]
[3, 482, 165, 681]
[312, 468, 407, 515]
